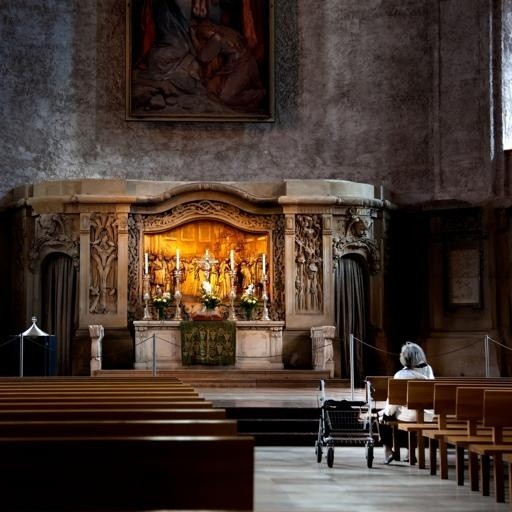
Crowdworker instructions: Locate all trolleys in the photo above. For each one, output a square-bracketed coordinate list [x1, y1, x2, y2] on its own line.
[315, 379, 382, 467]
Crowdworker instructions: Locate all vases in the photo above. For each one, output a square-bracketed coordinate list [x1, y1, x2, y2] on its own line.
[159, 306, 164, 320]
[248, 309, 253, 320]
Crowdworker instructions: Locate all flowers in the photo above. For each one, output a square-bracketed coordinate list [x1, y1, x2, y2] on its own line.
[201, 295, 221, 308]
[240, 294, 258, 309]
[151, 295, 170, 306]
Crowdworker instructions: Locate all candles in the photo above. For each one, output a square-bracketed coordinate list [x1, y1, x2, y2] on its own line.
[176, 250, 179, 271]
[145, 252, 148, 275]
[230, 250, 233, 268]
[262, 253, 266, 275]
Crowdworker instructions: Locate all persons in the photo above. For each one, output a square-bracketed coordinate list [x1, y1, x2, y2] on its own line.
[377, 341, 434, 465]
[333, 217, 380, 276]
[145, 255, 263, 301]
[189, 20, 266, 110]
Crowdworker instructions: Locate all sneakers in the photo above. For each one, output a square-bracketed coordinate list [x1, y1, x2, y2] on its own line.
[402, 455, 417, 462]
[383, 452, 395, 465]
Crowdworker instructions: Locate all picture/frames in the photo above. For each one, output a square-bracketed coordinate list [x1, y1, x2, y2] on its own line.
[444, 242, 484, 312]
[124, 0, 276, 121]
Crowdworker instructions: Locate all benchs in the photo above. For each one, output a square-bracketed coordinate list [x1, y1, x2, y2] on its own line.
[1, 376, 255, 512]
[365, 376, 512, 503]
